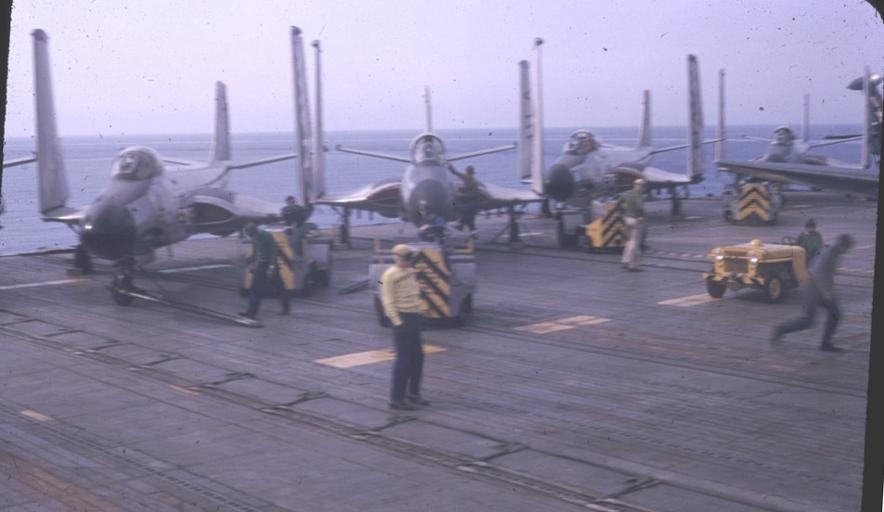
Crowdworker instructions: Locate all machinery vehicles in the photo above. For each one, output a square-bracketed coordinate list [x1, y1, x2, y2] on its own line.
[700, 236, 836, 300]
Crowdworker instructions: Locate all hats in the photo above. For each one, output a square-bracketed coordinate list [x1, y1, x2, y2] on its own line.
[391, 243, 413, 258]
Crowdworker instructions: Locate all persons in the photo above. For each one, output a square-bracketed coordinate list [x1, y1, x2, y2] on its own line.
[412, 198, 448, 249]
[791, 222, 819, 265]
[448, 165, 485, 256]
[379, 245, 431, 412]
[278, 193, 314, 259]
[770, 235, 860, 356]
[614, 177, 654, 273]
[238, 223, 294, 318]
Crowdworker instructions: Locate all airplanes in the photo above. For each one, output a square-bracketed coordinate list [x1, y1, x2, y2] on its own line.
[27, 25, 318, 304]
[716, 71, 884, 194]
[518, 55, 706, 251]
[285, 36, 549, 267]
[1, 152, 36, 174]
[707, 52, 867, 158]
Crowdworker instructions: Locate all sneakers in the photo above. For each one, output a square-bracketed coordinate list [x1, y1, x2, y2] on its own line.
[820, 342, 843, 353]
[387, 399, 416, 410]
[404, 391, 429, 406]
